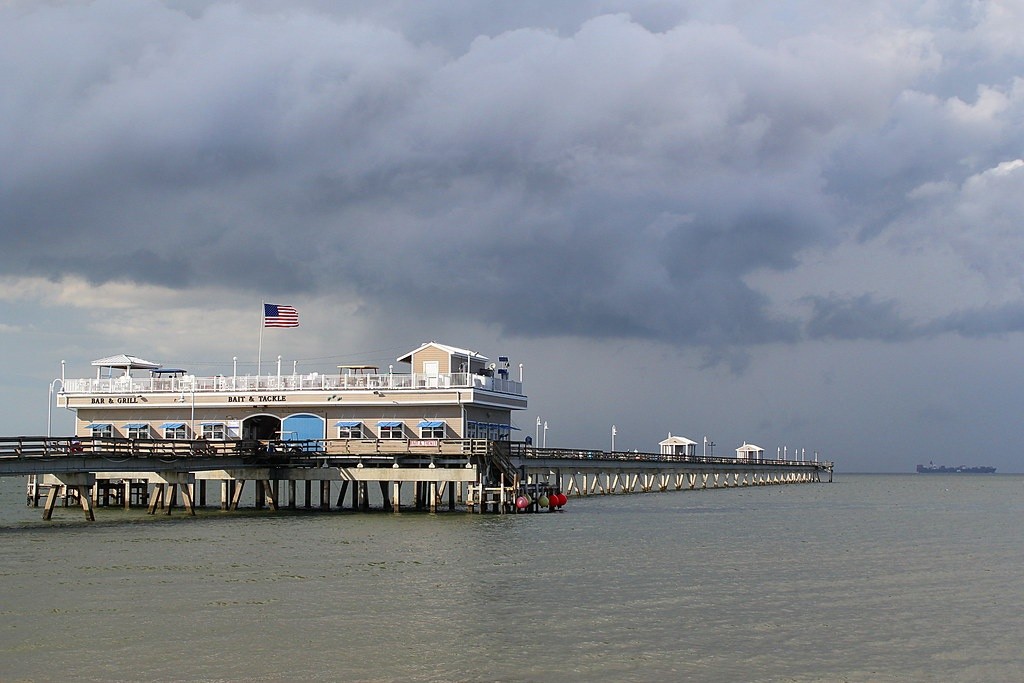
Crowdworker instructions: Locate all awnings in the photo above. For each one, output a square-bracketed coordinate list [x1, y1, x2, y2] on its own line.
[334, 421, 444, 427]
[84, 423, 223, 430]
[469, 421, 520, 431]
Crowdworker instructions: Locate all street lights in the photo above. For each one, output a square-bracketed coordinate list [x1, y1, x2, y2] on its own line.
[777, 446, 781, 464]
[801, 448, 805, 465]
[46, 378, 65, 454]
[62, 359, 66, 385]
[179, 387, 194, 440]
[794, 449, 798, 461]
[535, 416, 542, 457]
[276, 355, 283, 393]
[611, 424, 617, 458]
[519, 363, 524, 382]
[542, 420, 549, 453]
[703, 436, 708, 463]
[784, 445, 787, 464]
[233, 356, 237, 392]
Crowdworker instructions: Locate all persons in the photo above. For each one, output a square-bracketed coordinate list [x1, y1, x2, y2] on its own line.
[70, 435, 82, 457]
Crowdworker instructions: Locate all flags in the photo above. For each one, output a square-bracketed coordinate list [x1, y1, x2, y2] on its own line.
[263, 303, 300, 329]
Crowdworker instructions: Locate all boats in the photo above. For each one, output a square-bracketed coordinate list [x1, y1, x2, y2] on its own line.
[917, 460, 997, 474]
